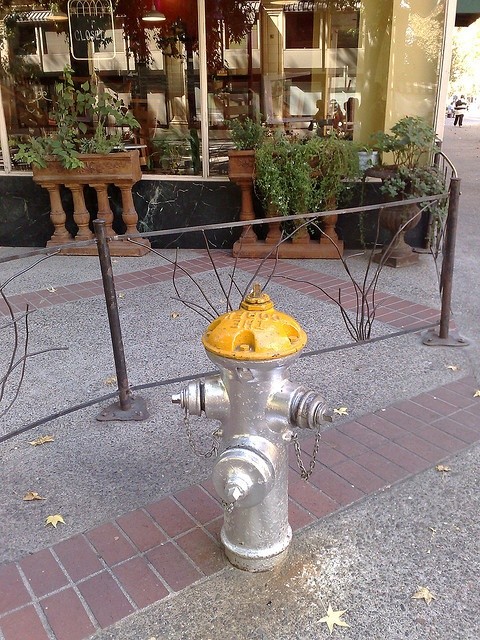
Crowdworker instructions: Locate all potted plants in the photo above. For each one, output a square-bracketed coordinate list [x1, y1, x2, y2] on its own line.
[227, 113, 358, 259]
[13, 64, 151, 257]
[359, 116, 449, 269]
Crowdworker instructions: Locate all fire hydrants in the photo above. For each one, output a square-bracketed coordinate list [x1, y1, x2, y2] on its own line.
[172, 281, 335, 574]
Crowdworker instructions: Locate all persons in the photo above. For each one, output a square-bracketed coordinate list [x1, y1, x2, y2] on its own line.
[451, 95, 468, 128]
